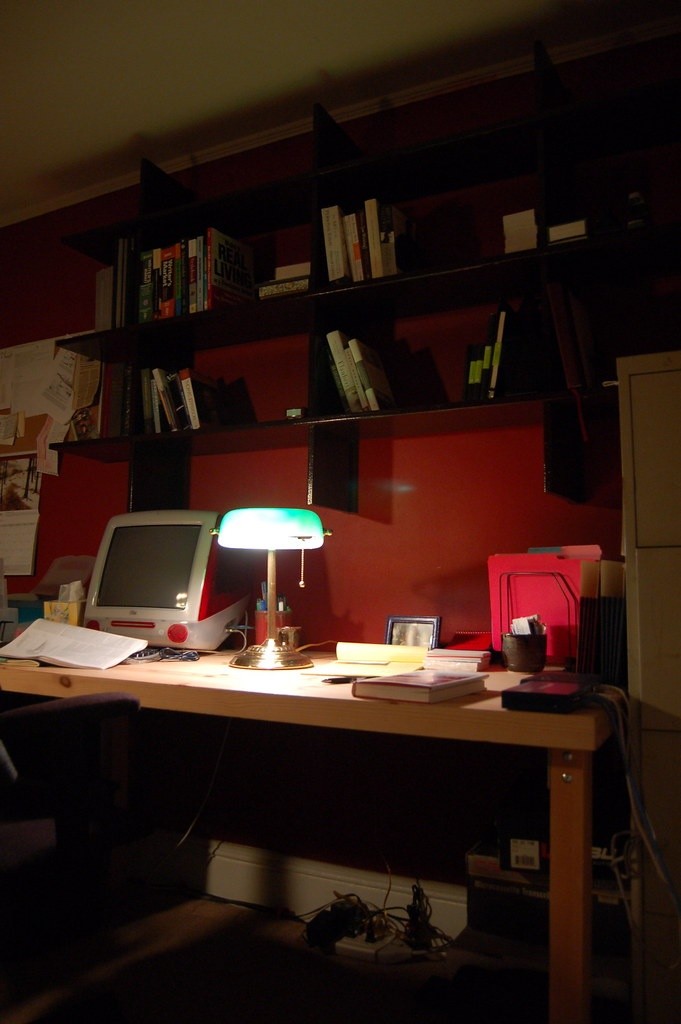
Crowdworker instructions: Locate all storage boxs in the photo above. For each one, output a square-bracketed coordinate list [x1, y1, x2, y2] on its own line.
[464, 822, 632, 960]
[446, 926, 631, 1024]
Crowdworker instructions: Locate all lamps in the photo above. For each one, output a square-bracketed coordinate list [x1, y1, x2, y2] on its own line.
[209, 508, 333, 670]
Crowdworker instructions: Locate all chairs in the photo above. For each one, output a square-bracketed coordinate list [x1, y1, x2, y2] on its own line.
[0, 694, 146, 927]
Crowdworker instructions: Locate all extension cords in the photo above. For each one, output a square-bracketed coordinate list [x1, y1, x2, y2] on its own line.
[334, 927, 412, 963]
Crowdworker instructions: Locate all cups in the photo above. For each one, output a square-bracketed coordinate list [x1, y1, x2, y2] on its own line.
[502, 632, 545, 672]
[255, 610, 290, 645]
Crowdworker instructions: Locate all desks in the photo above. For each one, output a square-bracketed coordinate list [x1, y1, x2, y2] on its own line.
[0, 649, 616, 1024]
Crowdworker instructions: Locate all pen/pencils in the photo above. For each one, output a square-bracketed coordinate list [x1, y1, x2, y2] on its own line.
[321, 676, 368, 684]
[255, 582, 290, 611]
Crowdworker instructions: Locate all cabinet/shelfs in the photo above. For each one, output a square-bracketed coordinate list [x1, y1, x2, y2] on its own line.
[49, 41, 681, 510]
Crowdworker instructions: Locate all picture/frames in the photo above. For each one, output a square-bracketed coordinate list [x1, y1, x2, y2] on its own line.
[385, 615, 442, 651]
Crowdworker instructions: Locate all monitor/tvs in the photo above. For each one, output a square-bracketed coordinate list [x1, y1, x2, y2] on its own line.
[85, 510, 253, 649]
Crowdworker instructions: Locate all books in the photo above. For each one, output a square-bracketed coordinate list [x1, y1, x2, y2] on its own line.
[93, 196, 589, 435]
[301, 545, 625, 704]
[0, 618, 148, 670]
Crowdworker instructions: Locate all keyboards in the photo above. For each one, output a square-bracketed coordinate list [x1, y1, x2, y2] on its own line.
[122, 649, 162, 663]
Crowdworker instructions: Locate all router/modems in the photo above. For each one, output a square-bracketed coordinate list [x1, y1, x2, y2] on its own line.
[501, 681, 590, 714]
[520, 673, 608, 698]
